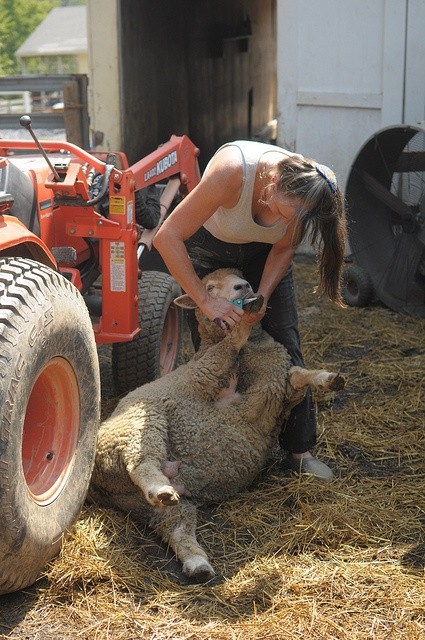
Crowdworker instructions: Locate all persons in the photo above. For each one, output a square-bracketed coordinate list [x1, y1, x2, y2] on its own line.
[151, 138, 348, 482]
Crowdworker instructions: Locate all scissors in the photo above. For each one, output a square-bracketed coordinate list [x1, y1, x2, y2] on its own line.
[204, 290, 258, 337]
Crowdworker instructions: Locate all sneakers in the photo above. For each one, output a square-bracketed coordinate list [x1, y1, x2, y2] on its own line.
[281, 456, 334, 482]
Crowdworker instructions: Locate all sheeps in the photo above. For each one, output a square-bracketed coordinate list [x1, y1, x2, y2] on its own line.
[86, 267, 348, 584]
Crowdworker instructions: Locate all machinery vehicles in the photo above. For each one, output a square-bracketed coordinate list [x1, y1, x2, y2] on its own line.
[0, 74, 201, 595]
